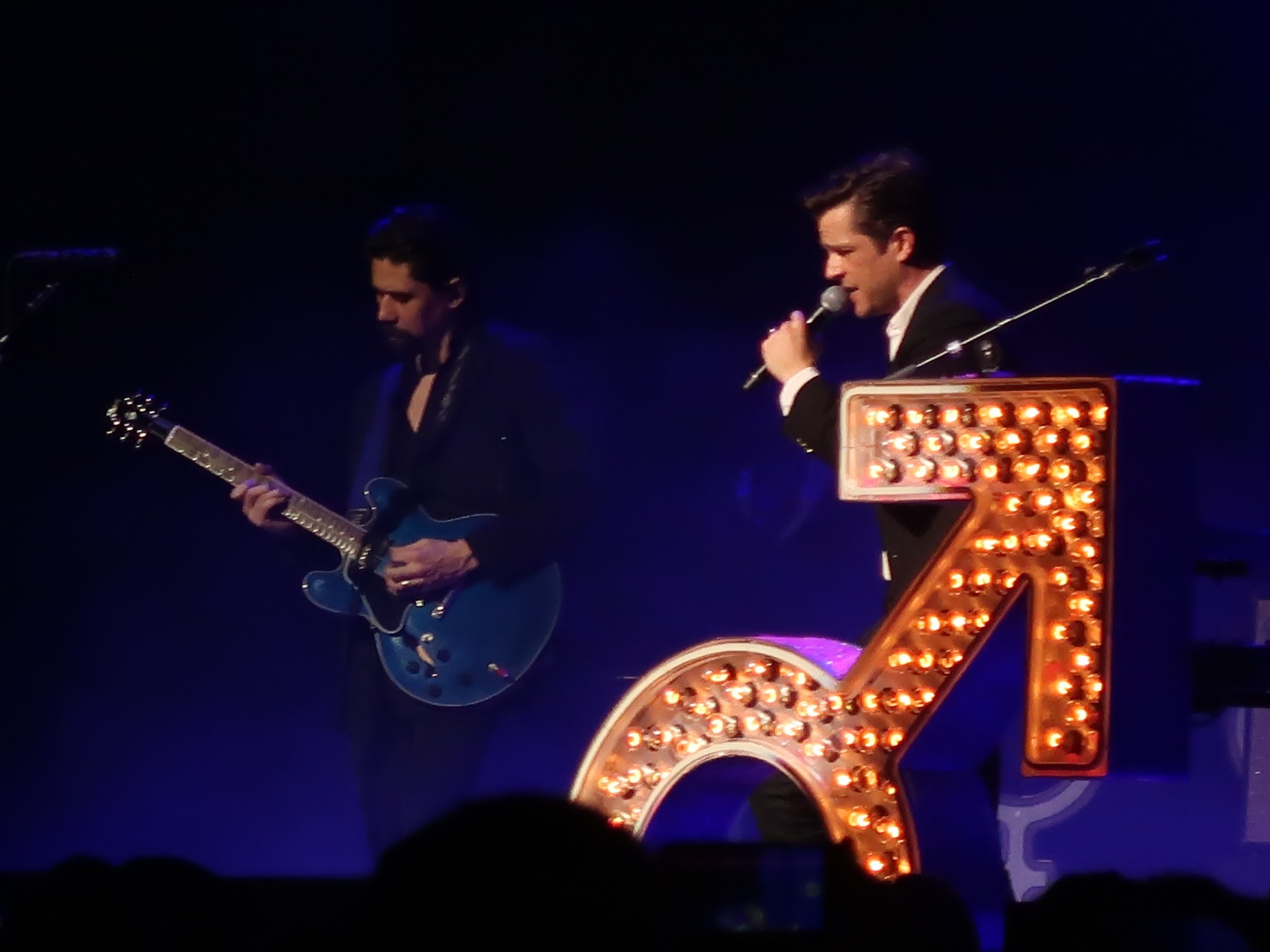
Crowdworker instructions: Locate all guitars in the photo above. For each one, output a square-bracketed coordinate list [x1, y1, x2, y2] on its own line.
[102, 393, 562, 708]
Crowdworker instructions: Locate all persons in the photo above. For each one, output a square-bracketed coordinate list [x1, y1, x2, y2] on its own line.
[758, 142, 1042, 906]
[230, 193, 596, 855]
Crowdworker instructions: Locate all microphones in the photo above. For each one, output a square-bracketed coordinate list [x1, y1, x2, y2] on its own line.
[914, 262, 1126, 372]
[743, 285, 849, 392]
